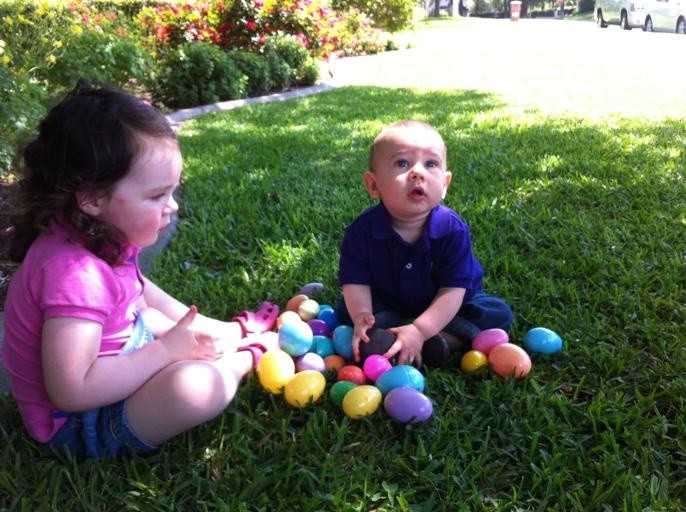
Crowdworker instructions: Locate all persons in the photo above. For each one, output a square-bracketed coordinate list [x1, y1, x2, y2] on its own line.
[330, 119, 514, 367]
[0, 76, 280, 465]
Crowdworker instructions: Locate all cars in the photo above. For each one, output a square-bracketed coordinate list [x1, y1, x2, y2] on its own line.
[593, 0, 686, 34]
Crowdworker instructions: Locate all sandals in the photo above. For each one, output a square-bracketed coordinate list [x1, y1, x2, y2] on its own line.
[361, 327, 395, 353]
[423, 332, 452, 364]
[231, 300, 279, 368]
